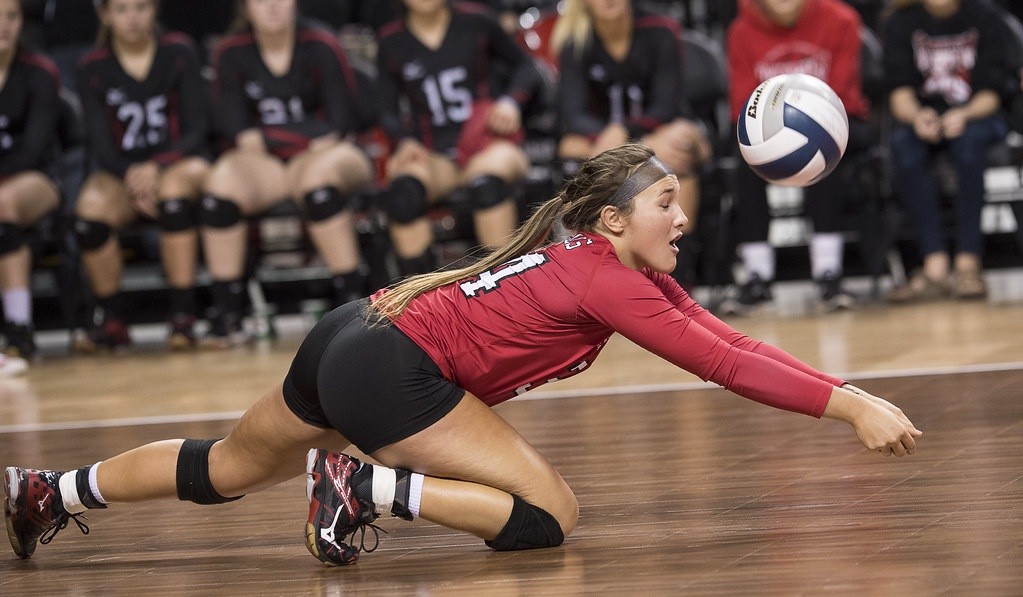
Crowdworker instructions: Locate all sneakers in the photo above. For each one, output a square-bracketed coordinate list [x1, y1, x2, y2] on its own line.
[303, 446, 390, 567]
[4, 466, 90, 559]
[170, 311, 197, 351]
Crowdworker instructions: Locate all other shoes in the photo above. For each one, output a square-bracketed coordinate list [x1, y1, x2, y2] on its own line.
[0, 322, 36, 359]
[954, 268, 988, 299]
[0, 353, 29, 376]
[812, 269, 856, 311]
[199, 311, 251, 349]
[70, 313, 135, 355]
[719, 271, 778, 318]
[889, 267, 949, 304]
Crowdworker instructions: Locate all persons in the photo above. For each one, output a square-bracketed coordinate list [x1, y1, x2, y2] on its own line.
[0, 145, 923, 567]
[0, 1, 1023, 353]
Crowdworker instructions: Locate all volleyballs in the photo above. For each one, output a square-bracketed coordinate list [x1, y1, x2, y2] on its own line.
[737, 73, 849, 187]
